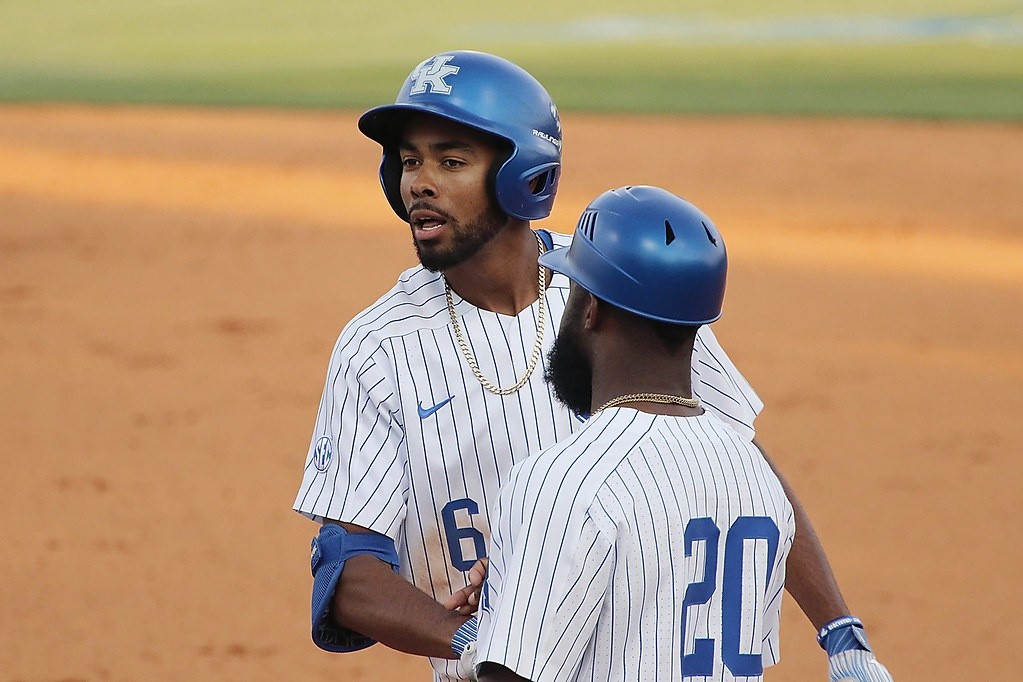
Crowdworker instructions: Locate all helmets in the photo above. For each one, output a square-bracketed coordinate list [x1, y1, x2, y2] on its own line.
[357, 51, 561, 220]
[537, 184, 728, 324]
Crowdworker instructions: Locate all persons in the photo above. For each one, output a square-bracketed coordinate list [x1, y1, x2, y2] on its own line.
[442, 182, 795, 682]
[293, 48, 901, 682]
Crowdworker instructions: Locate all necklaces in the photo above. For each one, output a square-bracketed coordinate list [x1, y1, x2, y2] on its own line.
[442, 230, 546, 396]
[588, 392, 702, 417]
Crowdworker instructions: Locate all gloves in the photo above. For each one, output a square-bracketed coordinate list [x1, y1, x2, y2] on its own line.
[822, 624, 894, 682]
